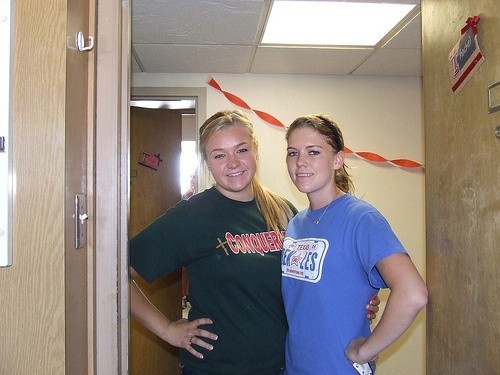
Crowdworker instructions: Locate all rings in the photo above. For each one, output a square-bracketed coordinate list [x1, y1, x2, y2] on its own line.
[190, 336, 195, 344]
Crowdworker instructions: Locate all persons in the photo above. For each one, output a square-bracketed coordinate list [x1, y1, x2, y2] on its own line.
[280, 114, 427, 375]
[129, 110, 380, 375]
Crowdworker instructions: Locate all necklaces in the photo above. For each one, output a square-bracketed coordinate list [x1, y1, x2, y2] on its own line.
[308, 188, 339, 225]
